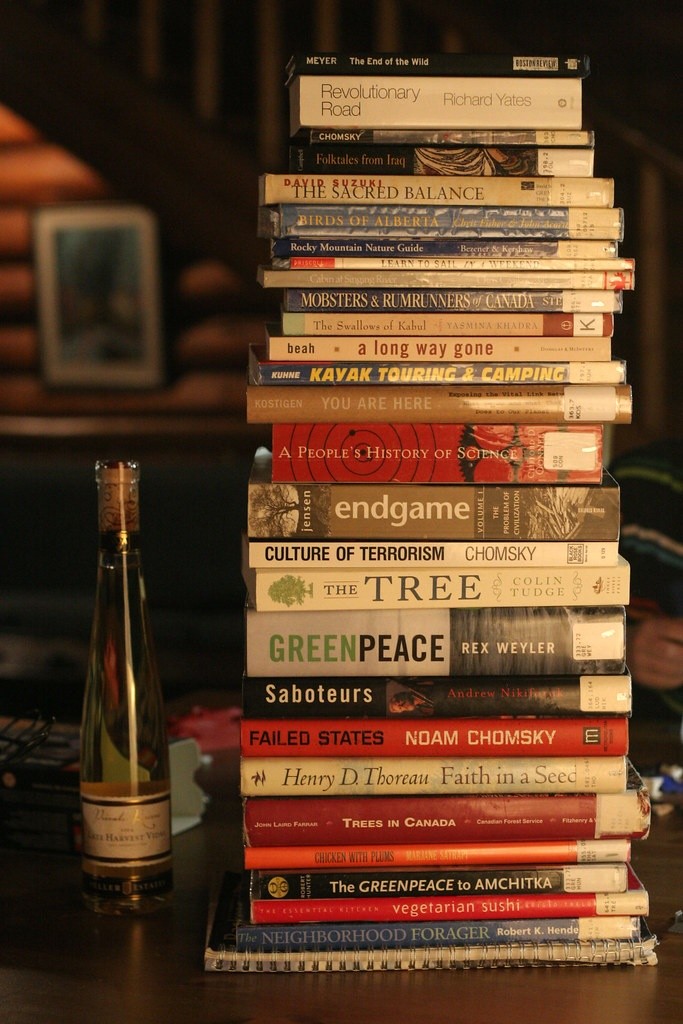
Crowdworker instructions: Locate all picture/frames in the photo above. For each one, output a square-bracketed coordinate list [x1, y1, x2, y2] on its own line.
[28, 199, 174, 396]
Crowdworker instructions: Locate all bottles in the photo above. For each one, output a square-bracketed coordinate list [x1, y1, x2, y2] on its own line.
[81, 458, 169, 915]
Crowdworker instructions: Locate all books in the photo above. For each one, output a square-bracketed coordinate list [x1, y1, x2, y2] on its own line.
[201, 51, 661, 976]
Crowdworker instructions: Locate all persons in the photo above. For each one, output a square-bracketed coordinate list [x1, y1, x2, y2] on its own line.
[609, 437, 683, 724]
[634, 722, 639, 738]
